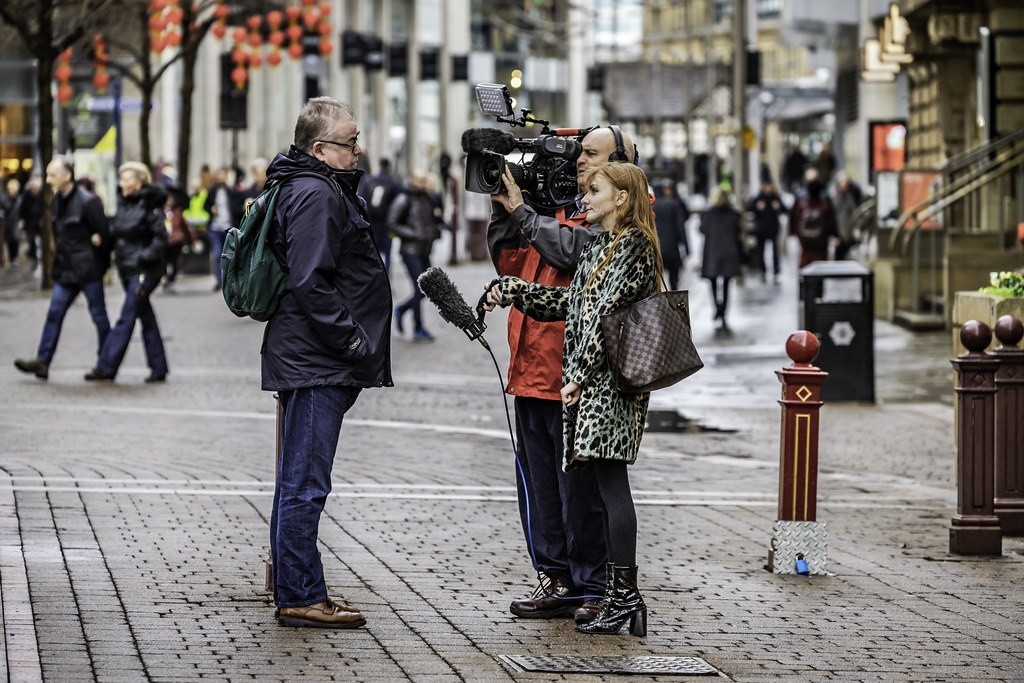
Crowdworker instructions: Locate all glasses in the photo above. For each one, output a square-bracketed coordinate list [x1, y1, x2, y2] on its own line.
[308, 134, 360, 147]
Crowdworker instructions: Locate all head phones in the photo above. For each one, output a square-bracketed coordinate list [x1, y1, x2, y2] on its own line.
[608, 125, 628, 163]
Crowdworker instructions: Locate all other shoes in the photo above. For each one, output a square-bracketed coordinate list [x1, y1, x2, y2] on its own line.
[146, 371, 167, 382]
[85, 368, 116, 381]
[416, 329, 430, 339]
[14, 360, 48, 378]
[396, 309, 403, 331]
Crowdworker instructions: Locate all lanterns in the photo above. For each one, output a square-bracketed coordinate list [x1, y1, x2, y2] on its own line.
[40, 1, 334, 110]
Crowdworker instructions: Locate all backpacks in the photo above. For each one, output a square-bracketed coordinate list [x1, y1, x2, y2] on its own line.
[222, 173, 344, 322]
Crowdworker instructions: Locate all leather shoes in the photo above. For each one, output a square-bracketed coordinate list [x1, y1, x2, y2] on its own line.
[275, 598, 366, 628]
[575, 598, 605, 622]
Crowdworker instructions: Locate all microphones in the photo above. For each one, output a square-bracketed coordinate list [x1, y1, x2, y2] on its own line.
[418, 266, 491, 351]
[461, 128, 535, 155]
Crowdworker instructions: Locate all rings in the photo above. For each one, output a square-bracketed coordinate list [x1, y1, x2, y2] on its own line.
[511, 182, 515, 186]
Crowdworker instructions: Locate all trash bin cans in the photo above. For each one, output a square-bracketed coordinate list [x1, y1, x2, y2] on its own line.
[797, 259, 877, 404]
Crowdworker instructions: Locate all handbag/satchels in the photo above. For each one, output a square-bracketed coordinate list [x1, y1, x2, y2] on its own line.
[601, 290, 704, 392]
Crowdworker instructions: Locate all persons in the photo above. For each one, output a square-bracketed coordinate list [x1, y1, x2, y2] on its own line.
[259, 95, 395, 630]
[354, 146, 461, 344]
[483, 160, 663, 639]
[651, 140, 865, 331]
[487, 125, 640, 620]
[1, 146, 266, 387]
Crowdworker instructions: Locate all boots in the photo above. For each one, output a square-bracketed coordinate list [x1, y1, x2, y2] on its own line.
[510, 572, 585, 617]
[575, 562, 647, 636]
